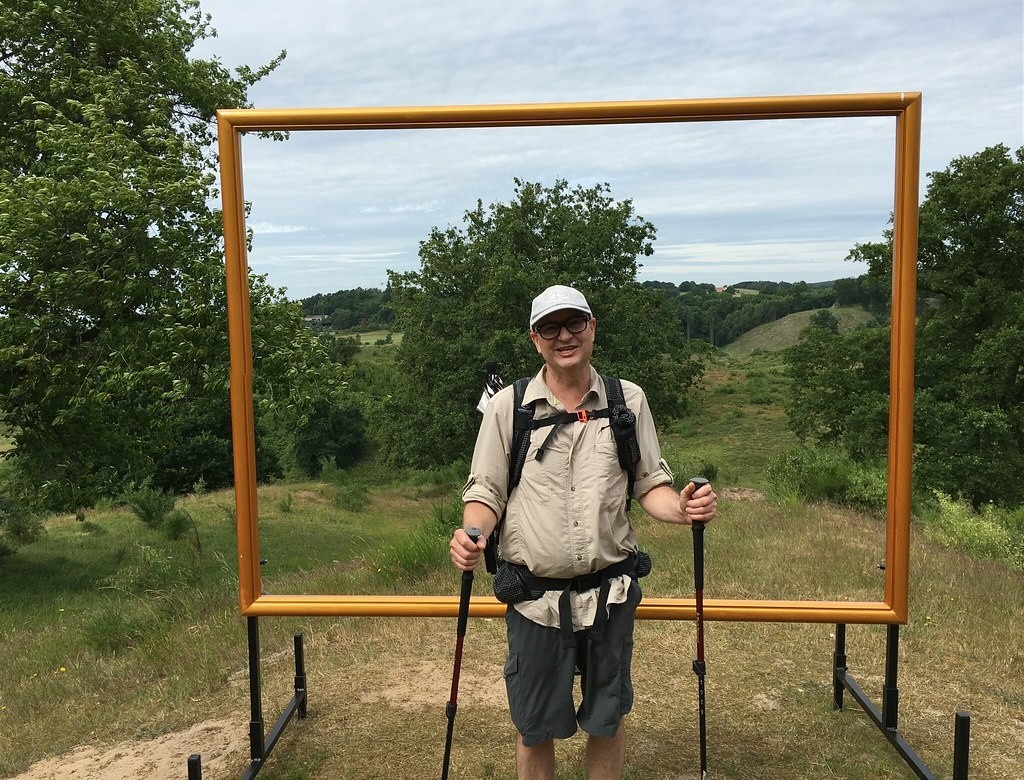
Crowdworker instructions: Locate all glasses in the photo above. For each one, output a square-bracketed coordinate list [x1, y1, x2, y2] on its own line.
[534, 315, 589, 341]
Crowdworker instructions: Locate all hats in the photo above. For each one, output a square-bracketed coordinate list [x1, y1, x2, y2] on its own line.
[530, 284, 593, 331]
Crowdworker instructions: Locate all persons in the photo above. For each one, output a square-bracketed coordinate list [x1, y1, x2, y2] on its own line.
[449, 285, 717, 780]
